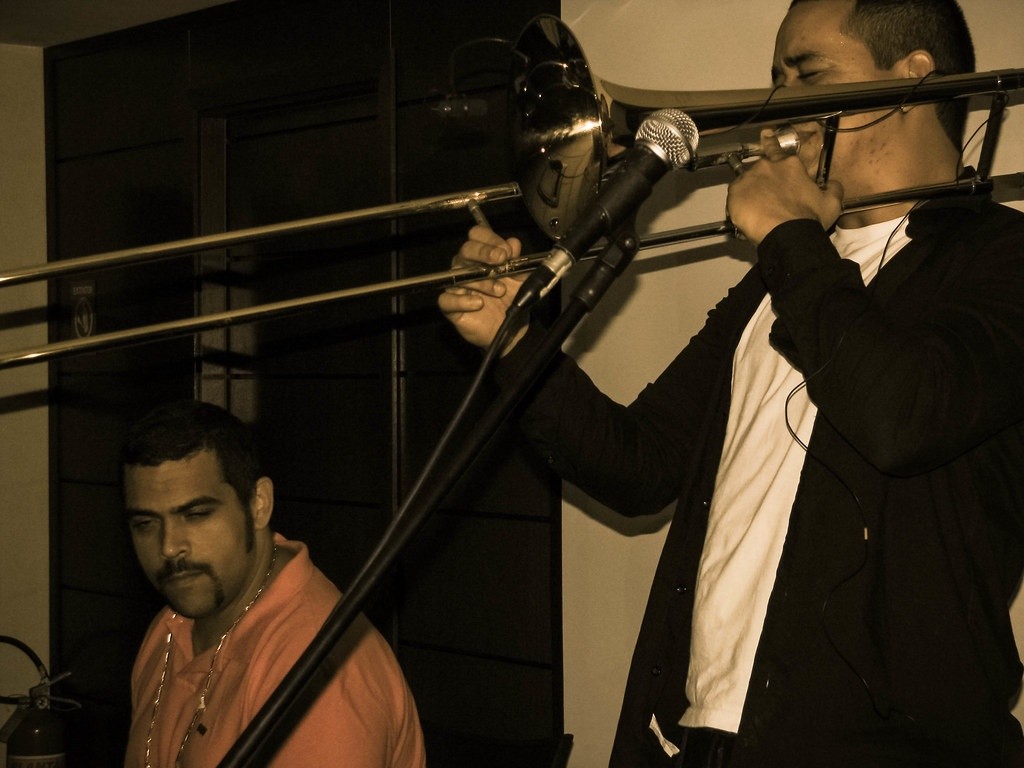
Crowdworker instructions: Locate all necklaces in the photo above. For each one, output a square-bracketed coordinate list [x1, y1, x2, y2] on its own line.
[144, 544, 279, 768]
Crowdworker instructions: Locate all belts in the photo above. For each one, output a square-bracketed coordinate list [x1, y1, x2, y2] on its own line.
[668, 726, 743, 768]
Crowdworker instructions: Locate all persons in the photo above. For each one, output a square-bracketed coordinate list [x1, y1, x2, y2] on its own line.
[123, 401, 427, 768]
[437, 0, 1024, 768]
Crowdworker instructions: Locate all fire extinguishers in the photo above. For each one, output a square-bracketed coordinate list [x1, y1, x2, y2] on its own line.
[0, 635, 83, 768]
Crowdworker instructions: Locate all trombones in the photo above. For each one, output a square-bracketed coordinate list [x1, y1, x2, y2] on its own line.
[0, 11, 1024, 375]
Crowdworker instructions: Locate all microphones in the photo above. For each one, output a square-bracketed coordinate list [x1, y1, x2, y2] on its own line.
[447, 37, 511, 118]
[505, 108, 699, 324]
